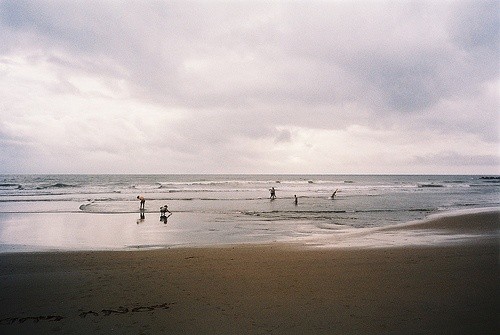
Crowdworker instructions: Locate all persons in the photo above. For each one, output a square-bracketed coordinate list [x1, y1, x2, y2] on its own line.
[269, 187, 277, 199]
[137, 196, 146, 209]
[331, 191, 337, 200]
[160, 205, 171, 216]
[293, 195, 298, 204]
